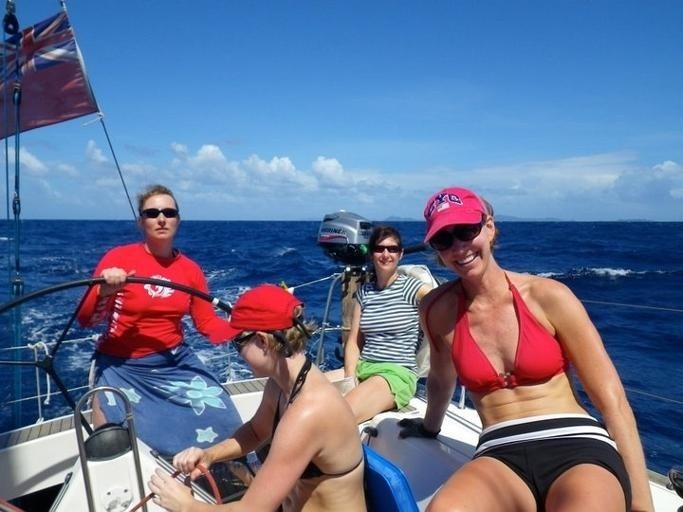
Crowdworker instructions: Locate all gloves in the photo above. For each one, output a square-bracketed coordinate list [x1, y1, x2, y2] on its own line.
[397, 417, 441, 440]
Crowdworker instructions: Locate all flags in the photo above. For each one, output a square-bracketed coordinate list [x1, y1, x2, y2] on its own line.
[0, 12, 99, 143]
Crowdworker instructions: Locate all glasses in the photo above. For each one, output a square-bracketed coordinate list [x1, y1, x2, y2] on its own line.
[372, 245, 402, 253]
[140, 207, 179, 217]
[427, 219, 484, 251]
[230, 331, 258, 350]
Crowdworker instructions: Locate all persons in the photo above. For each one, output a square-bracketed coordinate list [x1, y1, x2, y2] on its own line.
[147, 282, 367, 511]
[74, 181, 264, 502]
[340, 226, 437, 426]
[396, 185, 657, 512]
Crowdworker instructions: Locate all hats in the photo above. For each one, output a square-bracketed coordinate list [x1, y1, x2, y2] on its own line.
[422, 187, 486, 245]
[207, 282, 305, 345]
